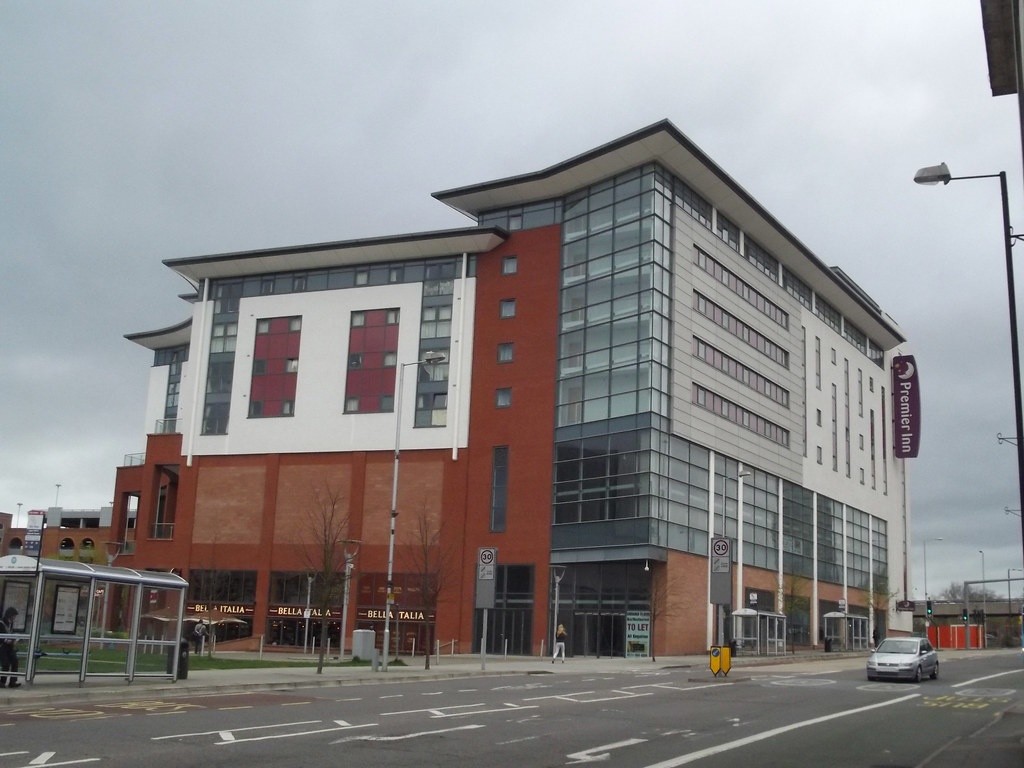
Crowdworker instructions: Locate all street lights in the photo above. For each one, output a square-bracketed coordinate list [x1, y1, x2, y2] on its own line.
[304, 569, 320, 653]
[55, 484, 61, 507]
[100, 540, 123, 652]
[731, 471, 750, 657]
[338, 538, 363, 659]
[382, 353, 443, 673]
[1008, 567, 1024, 645]
[548, 565, 567, 657]
[912, 162, 1024, 556]
[16, 503, 23, 528]
[924, 537, 943, 599]
[980, 549, 988, 650]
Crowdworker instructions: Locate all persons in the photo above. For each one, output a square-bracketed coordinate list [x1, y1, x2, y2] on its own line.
[195, 620, 206, 654]
[873, 626, 881, 649]
[0, 608, 21, 688]
[552, 624, 568, 663]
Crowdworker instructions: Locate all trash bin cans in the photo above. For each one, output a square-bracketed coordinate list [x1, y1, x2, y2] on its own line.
[825, 637, 832, 652]
[166, 637, 189, 680]
[728, 639, 736, 657]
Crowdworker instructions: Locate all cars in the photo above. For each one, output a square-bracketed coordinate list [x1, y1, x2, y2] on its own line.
[867, 635, 940, 682]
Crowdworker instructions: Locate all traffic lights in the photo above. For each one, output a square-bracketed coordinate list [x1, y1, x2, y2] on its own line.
[926, 600, 932, 614]
[962, 608, 968, 623]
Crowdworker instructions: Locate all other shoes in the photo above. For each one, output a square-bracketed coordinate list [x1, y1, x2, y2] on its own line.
[9, 682, 21, 687]
[562, 661, 564, 663]
[552, 660, 554, 664]
[0, 682, 6, 688]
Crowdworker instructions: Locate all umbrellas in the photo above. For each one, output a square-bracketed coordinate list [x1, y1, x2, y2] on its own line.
[141, 607, 246, 642]
